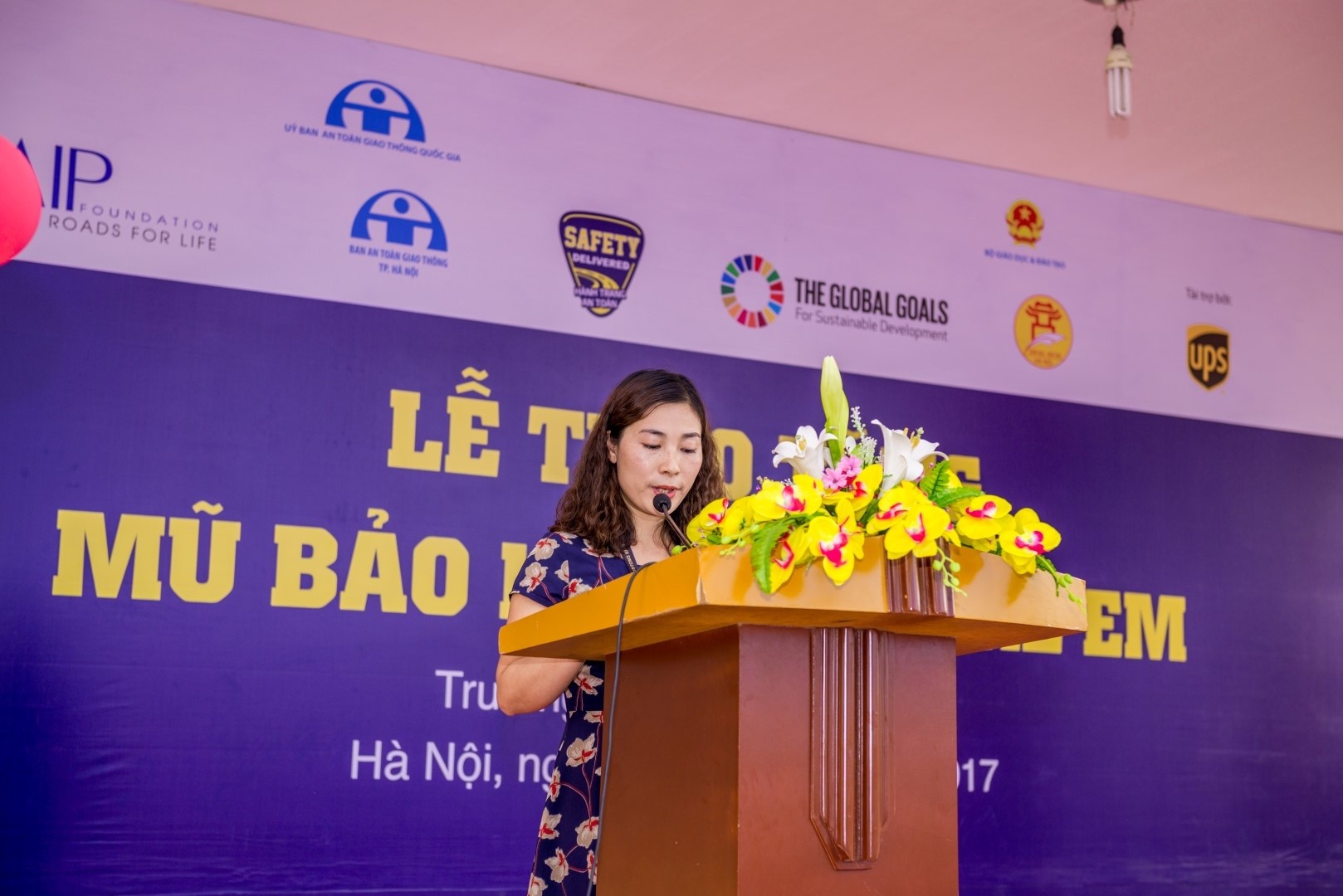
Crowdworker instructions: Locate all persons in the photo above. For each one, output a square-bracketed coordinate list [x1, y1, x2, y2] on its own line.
[497, 369, 727, 896]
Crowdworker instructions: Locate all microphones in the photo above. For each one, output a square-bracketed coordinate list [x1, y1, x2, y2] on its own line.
[654, 494, 694, 549]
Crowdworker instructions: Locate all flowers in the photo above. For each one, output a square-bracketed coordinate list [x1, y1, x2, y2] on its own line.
[673, 355, 1084, 607]
